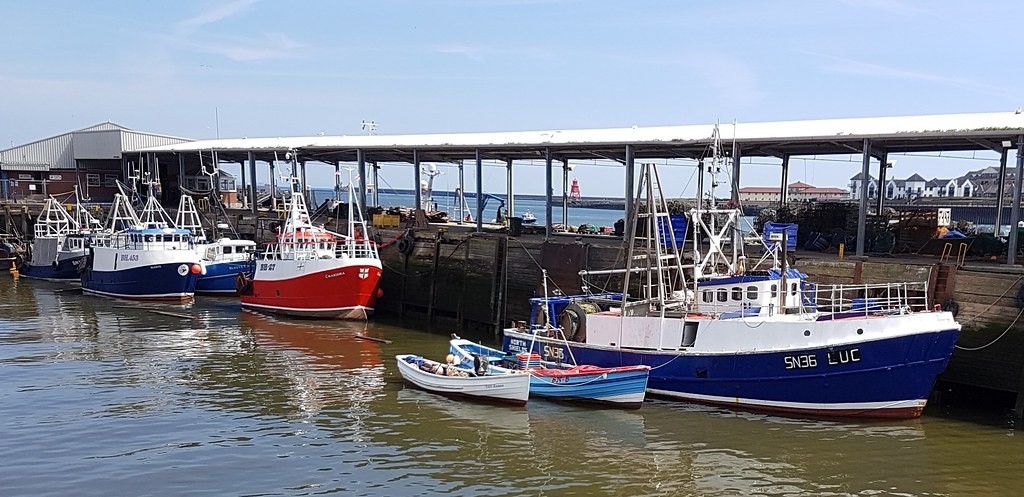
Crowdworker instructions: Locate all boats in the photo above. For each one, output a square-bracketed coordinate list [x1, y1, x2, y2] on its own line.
[395, 353, 532, 405]
[236, 150, 386, 321]
[16, 172, 255, 306]
[501, 115, 963, 421]
[445, 331, 653, 410]
[520, 209, 538, 224]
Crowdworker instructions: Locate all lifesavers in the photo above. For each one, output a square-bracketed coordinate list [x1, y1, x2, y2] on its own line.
[942, 301, 959, 316]
[52, 260, 60, 271]
[724, 200, 739, 218]
[1016, 284, 1024, 312]
[24, 263, 30, 272]
[396, 235, 414, 255]
[269, 223, 278, 232]
[369, 234, 382, 250]
